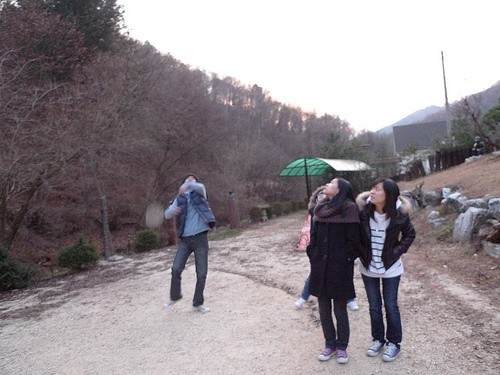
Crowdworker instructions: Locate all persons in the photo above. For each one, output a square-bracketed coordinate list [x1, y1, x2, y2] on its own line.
[306, 178, 361, 364]
[294, 186, 358, 310]
[472, 136, 485, 156]
[355, 177, 416, 361]
[164, 173, 216, 313]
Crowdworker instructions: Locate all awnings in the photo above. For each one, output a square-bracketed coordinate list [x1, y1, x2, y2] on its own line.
[279, 157, 372, 204]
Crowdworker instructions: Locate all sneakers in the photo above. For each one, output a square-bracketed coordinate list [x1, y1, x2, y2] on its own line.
[347, 301, 358, 311]
[164, 297, 181, 307]
[295, 297, 306, 308]
[318, 348, 338, 360]
[382, 342, 401, 361]
[367, 340, 384, 356]
[195, 304, 210, 312]
[337, 349, 348, 363]
